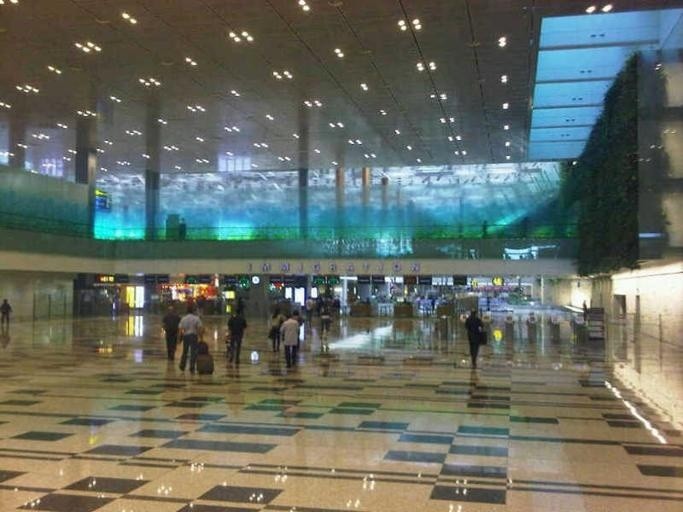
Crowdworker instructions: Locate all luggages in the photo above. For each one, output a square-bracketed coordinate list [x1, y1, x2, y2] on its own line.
[196, 334, 214, 375]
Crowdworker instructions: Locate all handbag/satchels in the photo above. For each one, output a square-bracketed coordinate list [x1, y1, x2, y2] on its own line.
[479, 331, 487, 345]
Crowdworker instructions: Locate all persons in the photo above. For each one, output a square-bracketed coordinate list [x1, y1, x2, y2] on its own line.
[0, 297, 13, 329]
[159, 291, 344, 374]
[463, 306, 488, 369]
